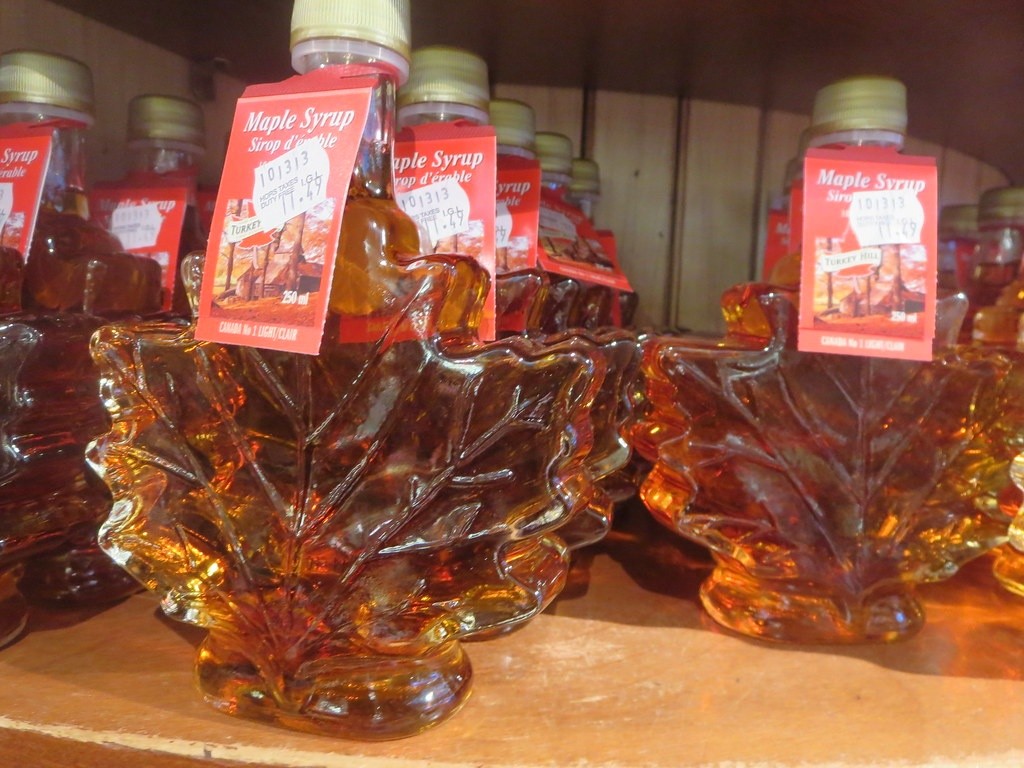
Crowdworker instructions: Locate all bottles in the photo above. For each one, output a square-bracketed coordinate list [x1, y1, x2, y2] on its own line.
[88, 2, 644, 742]
[621, 80, 1024, 643]
[0, 47, 157, 651]
[101, 96, 228, 332]
